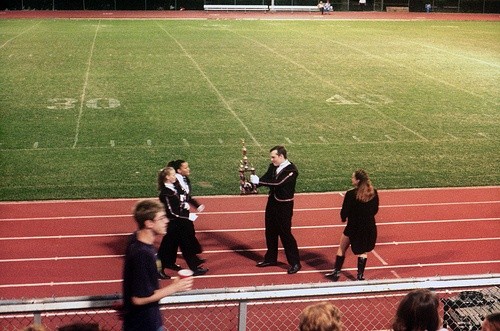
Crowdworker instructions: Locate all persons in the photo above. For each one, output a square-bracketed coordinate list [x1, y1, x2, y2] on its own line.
[123, 200, 194, 331]
[162, 160, 205, 271]
[324, 0, 331, 15]
[391, 289, 450, 331]
[155, 167, 209, 279]
[317, 1, 325, 14]
[358, 0, 366, 11]
[425, 1, 432, 14]
[298, 303, 344, 331]
[325, 168, 380, 281]
[246, 146, 301, 274]
[481, 312, 500, 331]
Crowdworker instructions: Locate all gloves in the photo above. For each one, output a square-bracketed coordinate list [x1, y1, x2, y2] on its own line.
[196, 204, 205, 212]
[189, 212, 198, 221]
[244, 182, 251, 187]
[249, 174, 260, 184]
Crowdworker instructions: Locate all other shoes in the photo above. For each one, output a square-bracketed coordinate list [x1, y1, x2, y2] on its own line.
[256, 260, 277, 267]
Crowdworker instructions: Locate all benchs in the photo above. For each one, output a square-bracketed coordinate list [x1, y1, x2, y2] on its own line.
[269, 6, 333, 14]
[203, 5, 269, 14]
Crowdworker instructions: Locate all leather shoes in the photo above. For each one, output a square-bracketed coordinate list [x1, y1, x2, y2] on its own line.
[193, 269, 208, 275]
[288, 263, 301, 274]
[166, 263, 181, 269]
[189, 259, 207, 269]
[157, 269, 171, 279]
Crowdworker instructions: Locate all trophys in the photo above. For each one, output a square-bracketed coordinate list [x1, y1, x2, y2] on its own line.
[238, 139, 258, 195]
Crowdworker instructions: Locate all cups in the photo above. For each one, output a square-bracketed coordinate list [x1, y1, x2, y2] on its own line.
[178, 270, 193, 280]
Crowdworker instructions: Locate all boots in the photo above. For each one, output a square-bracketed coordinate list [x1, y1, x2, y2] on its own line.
[357, 257, 367, 280]
[325, 254, 345, 281]
